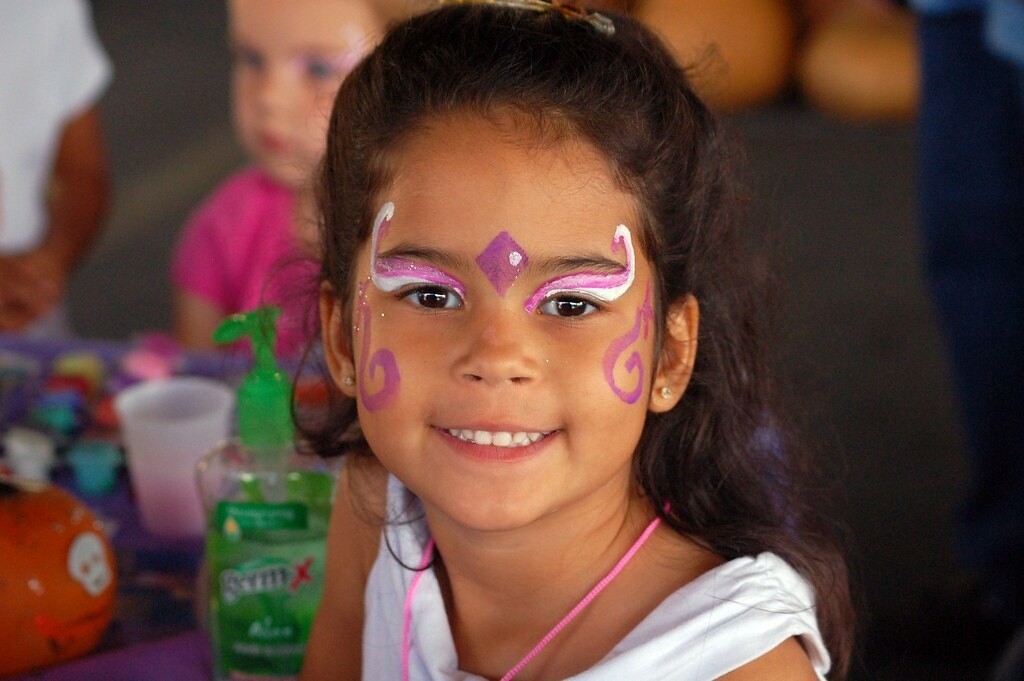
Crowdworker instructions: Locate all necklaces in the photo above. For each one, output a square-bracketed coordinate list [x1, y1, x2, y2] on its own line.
[400, 501, 672, 681]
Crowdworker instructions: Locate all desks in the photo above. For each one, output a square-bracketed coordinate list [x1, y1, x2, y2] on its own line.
[1, 336, 345, 681]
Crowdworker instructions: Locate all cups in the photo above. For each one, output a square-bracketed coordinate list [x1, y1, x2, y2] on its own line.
[114, 377, 230, 537]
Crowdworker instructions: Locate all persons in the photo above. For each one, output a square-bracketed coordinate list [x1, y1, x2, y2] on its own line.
[294, 0, 856, 681]
[0, 0, 119, 348]
[172, 0, 454, 369]
[909, 0, 1024, 680]
